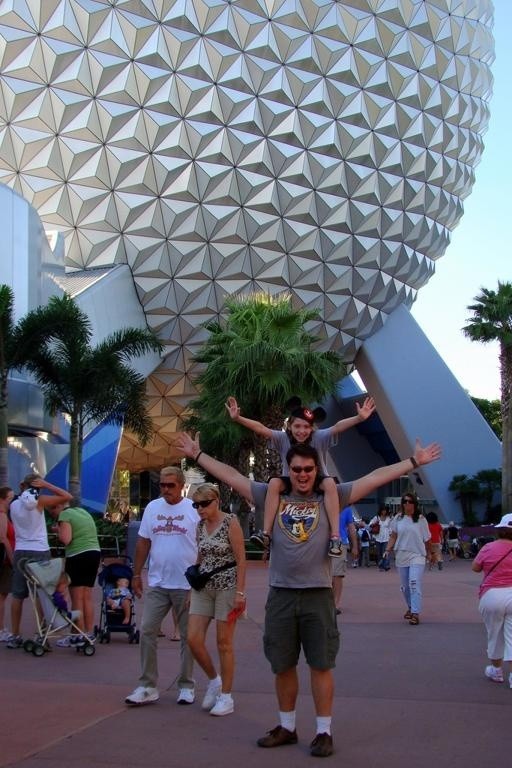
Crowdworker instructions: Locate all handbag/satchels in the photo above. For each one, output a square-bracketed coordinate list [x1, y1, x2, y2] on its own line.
[370, 522, 380, 534]
[185, 564, 210, 591]
[378, 552, 391, 571]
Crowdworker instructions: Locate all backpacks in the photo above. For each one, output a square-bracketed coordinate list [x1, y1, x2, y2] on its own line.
[360, 529, 369, 542]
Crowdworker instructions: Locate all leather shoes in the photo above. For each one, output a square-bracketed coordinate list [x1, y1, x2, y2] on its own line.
[257, 724, 297, 748]
[311, 732, 333, 757]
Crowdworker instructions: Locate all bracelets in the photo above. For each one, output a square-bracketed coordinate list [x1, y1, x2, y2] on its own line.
[410, 456, 418, 468]
[133, 575, 141, 579]
[236, 591, 244, 596]
[195, 450, 203, 462]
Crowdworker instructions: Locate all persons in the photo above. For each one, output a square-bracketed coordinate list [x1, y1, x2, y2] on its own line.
[330, 477, 459, 625]
[0, 486, 14, 641]
[44, 500, 101, 647]
[175, 430, 443, 758]
[157, 607, 179, 641]
[224, 395, 377, 559]
[105, 578, 132, 624]
[185, 482, 249, 717]
[125, 466, 202, 706]
[8, 472, 74, 648]
[471, 513, 512, 690]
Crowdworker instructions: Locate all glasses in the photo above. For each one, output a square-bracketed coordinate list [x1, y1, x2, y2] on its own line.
[292, 466, 314, 473]
[191, 499, 216, 508]
[403, 499, 412, 504]
[159, 482, 175, 488]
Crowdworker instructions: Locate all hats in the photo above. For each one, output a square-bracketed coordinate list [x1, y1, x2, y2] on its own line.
[493, 513, 512, 528]
[21, 487, 40, 510]
[285, 396, 327, 425]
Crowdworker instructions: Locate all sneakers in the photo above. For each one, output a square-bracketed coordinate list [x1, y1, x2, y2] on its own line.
[327, 539, 343, 558]
[201, 679, 222, 709]
[250, 531, 271, 550]
[438, 560, 444, 570]
[485, 665, 503, 682]
[404, 609, 419, 624]
[125, 687, 159, 705]
[209, 696, 233, 716]
[56, 633, 95, 647]
[0, 629, 26, 648]
[34, 635, 53, 651]
[176, 688, 194, 704]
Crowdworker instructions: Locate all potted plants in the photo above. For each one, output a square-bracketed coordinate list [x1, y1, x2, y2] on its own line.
[448, 469, 503, 542]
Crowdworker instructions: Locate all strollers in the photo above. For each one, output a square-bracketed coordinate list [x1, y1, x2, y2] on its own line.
[92, 555, 141, 646]
[15, 557, 98, 657]
